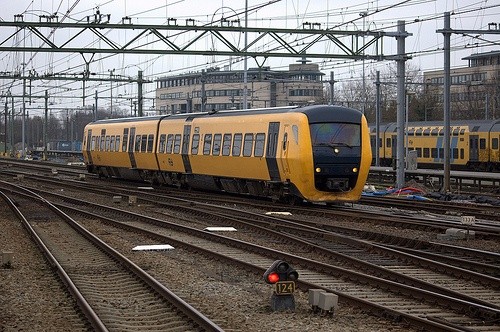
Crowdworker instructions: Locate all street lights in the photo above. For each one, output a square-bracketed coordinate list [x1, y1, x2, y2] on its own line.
[405, 91, 416, 169]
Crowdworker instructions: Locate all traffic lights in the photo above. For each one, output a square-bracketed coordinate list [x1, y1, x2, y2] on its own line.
[263, 259, 299, 284]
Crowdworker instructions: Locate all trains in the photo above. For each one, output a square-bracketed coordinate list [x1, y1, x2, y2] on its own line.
[79, 103, 374, 208]
[367, 120, 500, 174]
